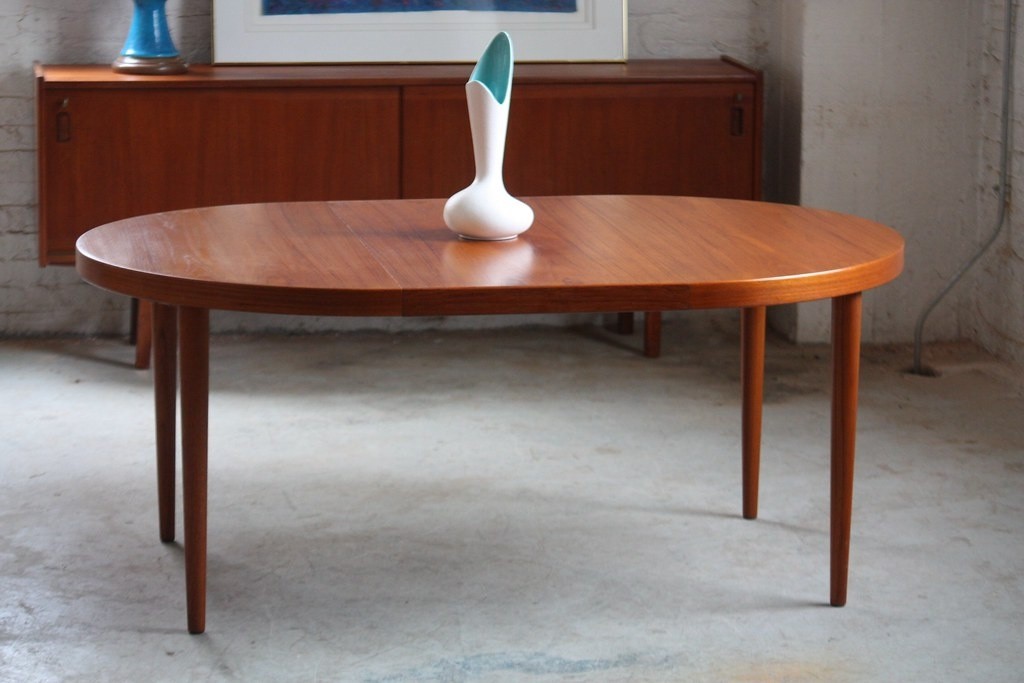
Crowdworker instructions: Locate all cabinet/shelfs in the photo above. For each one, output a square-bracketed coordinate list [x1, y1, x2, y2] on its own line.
[32, 56, 763, 369]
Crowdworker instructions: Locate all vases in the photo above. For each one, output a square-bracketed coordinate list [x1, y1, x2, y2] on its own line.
[442, 30, 533, 242]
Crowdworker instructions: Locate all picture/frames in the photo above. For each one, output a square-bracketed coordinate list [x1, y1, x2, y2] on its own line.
[213, 0, 629, 65]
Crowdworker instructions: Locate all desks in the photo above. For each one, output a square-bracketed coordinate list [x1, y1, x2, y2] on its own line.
[73, 194, 905, 634]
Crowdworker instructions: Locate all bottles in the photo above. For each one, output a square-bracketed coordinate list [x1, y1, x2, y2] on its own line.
[442, 30, 536, 241]
